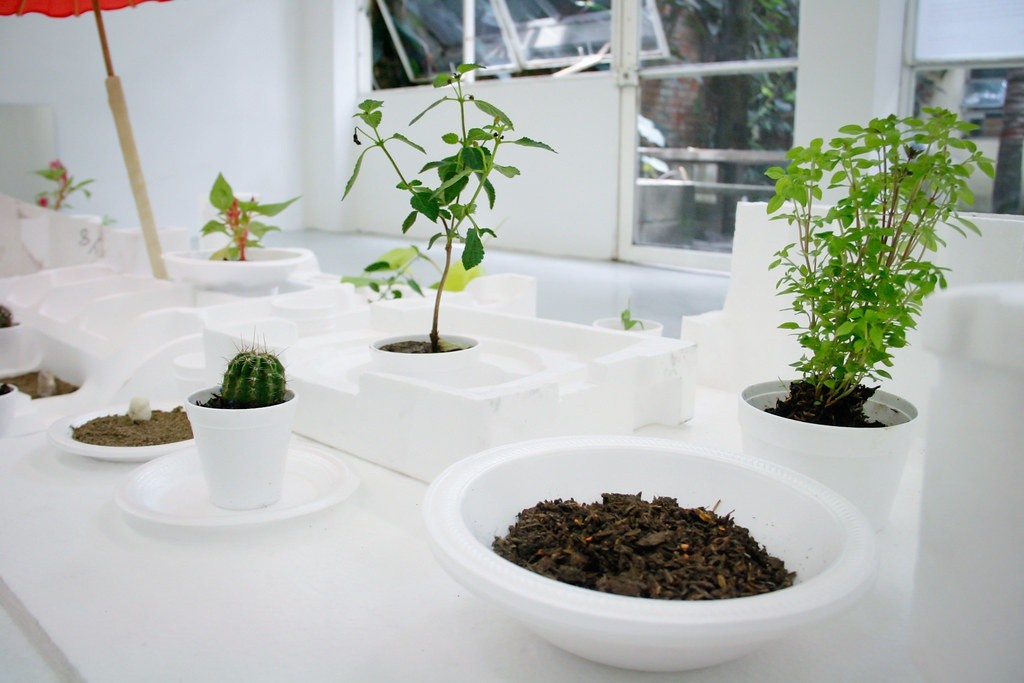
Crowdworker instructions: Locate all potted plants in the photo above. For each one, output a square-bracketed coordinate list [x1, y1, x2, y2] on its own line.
[186, 337, 298, 505]
[723, 109, 1000, 506]
[340, 58, 560, 391]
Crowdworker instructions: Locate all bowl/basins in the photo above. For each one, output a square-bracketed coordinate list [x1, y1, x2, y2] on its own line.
[420, 434, 879, 673]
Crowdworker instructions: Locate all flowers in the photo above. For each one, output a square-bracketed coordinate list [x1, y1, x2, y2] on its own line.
[198, 171, 301, 263]
[33, 161, 97, 212]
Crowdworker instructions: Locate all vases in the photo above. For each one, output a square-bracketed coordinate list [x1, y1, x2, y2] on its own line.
[192, 245, 325, 297]
